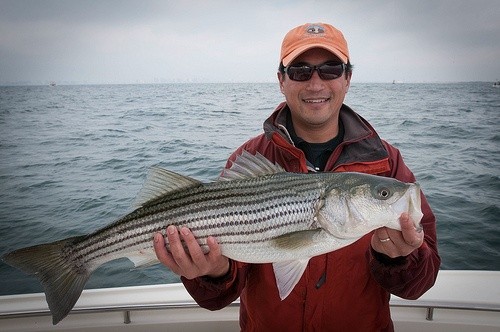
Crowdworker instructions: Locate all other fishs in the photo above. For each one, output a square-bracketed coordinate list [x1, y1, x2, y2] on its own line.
[0, 148, 424, 324]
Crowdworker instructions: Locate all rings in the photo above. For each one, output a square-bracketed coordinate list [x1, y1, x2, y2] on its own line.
[380, 237, 391, 242]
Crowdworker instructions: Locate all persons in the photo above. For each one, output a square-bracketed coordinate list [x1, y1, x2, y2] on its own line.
[154, 23, 441, 332]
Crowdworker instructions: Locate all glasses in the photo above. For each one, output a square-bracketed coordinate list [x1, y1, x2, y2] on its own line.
[284, 61, 347, 82]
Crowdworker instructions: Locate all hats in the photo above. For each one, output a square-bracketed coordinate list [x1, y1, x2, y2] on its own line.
[280, 23, 349, 67]
